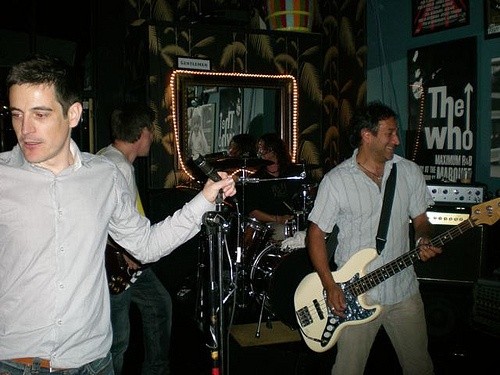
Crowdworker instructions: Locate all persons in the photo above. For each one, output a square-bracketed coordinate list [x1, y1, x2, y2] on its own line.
[94, 103, 174, 375]
[305, 101, 445, 375]
[227, 132, 252, 157]
[243, 133, 305, 223]
[0, 57, 237, 375]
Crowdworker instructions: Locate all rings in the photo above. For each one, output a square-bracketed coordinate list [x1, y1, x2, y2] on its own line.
[331, 306, 336, 311]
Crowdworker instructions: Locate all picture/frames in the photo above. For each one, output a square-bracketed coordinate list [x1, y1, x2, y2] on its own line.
[483, 0, 500, 40]
[412, 0, 470, 37]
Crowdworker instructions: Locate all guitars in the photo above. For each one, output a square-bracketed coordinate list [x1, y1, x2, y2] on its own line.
[106, 234, 136, 294]
[294, 198, 500, 353]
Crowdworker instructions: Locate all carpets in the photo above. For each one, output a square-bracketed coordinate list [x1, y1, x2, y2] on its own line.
[227, 322, 302, 348]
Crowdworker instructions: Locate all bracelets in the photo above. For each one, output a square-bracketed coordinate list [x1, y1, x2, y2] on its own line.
[415, 237, 422, 248]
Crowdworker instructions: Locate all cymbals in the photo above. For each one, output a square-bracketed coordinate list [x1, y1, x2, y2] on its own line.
[177, 184, 234, 208]
[280, 163, 319, 171]
[218, 158, 273, 171]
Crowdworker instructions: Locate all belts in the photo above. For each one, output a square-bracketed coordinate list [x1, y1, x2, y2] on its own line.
[11, 358, 50, 369]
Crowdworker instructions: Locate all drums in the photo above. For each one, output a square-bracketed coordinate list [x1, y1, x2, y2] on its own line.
[220, 211, 271, 267]
[248, 240, 336, 330]
[292, 185, 336, 239]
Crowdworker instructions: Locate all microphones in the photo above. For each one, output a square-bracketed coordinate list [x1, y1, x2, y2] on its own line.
[192, 153, 238, 201]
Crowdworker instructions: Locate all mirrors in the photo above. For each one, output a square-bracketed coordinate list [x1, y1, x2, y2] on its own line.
[177, 73, 292, 183]
